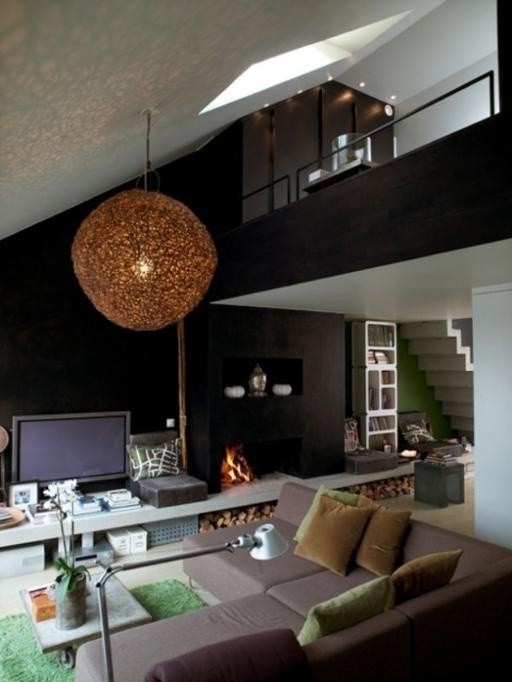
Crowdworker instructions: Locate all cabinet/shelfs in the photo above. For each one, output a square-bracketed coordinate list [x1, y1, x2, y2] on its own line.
[353, 320, 401, 451]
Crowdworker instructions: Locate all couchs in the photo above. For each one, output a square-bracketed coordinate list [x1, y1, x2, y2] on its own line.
[67, 482, 512, 682]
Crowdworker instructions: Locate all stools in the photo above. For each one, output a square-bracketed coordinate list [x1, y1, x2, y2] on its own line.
[414, 460, 465, 509]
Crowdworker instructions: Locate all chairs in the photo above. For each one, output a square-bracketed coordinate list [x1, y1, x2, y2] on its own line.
[127, 430, 207, 508]
[398, 411, 463, 457]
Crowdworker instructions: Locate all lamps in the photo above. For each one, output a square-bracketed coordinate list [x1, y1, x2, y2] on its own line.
[95, 523, 291, 682]
[70, 109, 220, 333]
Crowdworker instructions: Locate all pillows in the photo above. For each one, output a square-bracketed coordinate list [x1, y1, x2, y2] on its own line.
[401, 428, 437, 445]
[122, 438, 186, 480]
[293, 484, 465, 647]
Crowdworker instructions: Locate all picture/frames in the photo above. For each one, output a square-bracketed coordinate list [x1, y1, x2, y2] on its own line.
[6, 480, 40, 513]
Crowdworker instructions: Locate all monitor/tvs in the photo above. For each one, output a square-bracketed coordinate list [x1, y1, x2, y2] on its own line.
[11, 410, 131, 488]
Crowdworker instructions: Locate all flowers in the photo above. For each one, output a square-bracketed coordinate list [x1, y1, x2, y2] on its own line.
[43, 478, 92, 602]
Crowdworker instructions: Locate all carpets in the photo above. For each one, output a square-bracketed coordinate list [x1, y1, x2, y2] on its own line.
[0, 578, 209, 682]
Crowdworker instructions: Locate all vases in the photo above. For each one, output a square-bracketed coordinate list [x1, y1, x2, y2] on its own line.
[52, 571, 90, 629]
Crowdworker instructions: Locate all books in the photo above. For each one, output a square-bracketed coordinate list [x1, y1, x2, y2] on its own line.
[423, 451, 457, 465]
[369, 325, 393, 451]
[26, 488, 141, 525]
